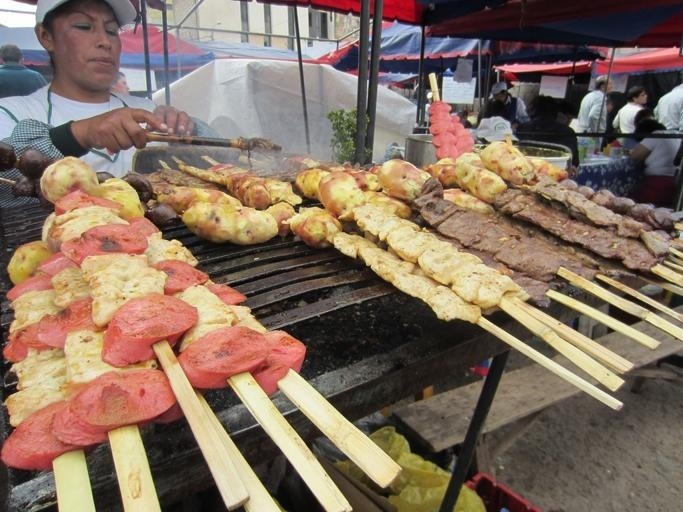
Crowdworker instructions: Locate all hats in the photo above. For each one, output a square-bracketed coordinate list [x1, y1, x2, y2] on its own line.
[36, 0, 139, 28]
[492, 81, 508, 94]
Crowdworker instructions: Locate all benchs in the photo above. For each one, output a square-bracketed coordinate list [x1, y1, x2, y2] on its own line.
[390, 303, 682, 484]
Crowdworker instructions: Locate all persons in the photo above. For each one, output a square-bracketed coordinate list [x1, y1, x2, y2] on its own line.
[612, 85, 648, 141]
[515, 95, 579, 168]
[483, 82, 531, 135]
[0, 44, 48, 99]
[633, 109, 655, 127]
[577, 75, 612, 156]
[652, 83, 683, 131]
[0, 0, 198, 208]
[630, 119, 683, 208]
